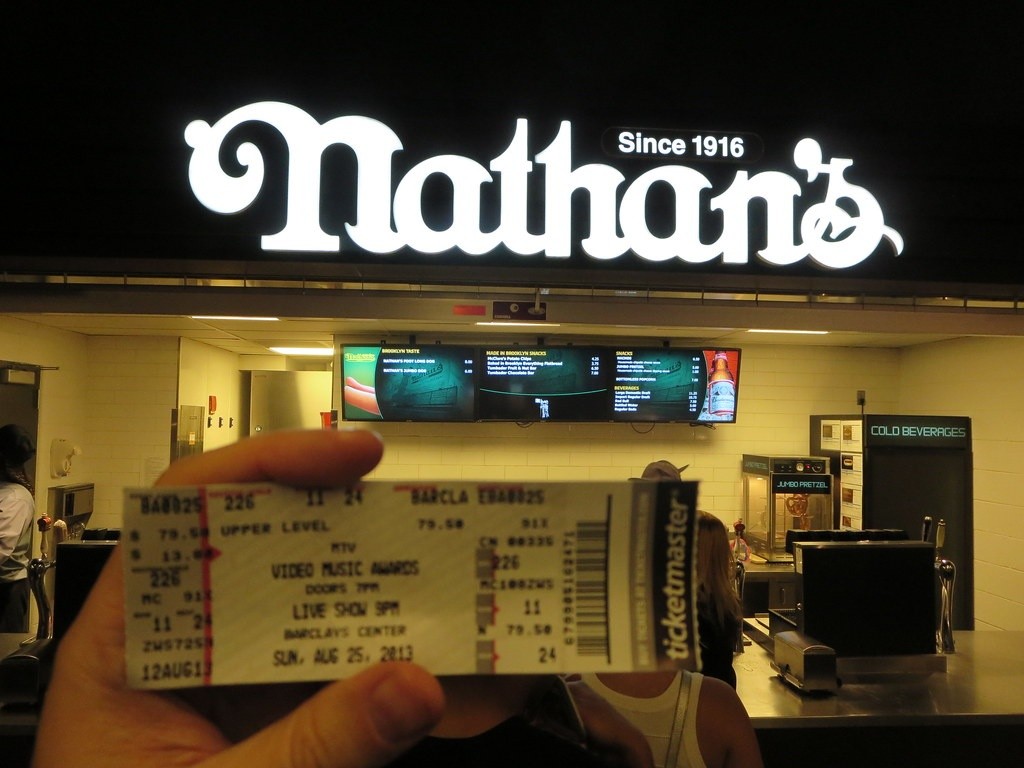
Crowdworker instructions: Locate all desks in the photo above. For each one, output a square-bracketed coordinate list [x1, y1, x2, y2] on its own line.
[53, 539, 119, 639]
[731, 560, 1024, 729]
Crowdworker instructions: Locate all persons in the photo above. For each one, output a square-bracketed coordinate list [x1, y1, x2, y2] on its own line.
[30, 431, 763, 767]
[0, 423, 35, 634]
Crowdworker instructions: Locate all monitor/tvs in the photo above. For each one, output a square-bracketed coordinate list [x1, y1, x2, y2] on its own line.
[334, 336, 741, 424]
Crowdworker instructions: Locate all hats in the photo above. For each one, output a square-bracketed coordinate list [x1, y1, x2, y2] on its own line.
[628, 460, 689, 481]
